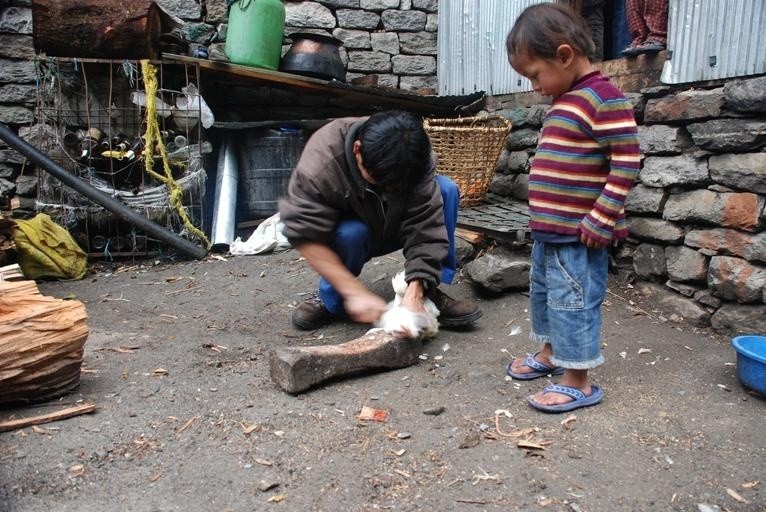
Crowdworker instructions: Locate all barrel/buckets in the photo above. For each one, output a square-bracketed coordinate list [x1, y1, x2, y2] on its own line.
[224, 0, 285, 71]
[240, 128, 306, 218]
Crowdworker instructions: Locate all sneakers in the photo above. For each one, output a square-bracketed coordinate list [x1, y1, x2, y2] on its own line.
[429, 286, 483, 328]
[292, 292, 338, 330]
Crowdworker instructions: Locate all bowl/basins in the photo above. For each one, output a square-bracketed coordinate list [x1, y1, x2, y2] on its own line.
[729, 334, 766, 397]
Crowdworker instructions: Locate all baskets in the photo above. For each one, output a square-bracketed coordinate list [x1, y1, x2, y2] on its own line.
[421, 111, 512, 208]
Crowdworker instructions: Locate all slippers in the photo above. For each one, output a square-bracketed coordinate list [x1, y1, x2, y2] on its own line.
[622, 38, 666, 53]
[526, 383, 605, 414]
[505, 348, 566, 379]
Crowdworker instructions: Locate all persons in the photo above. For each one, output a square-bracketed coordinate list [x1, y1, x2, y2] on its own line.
[277, 108, 483, 330]
[504, 1, 644, 413]
[581, 0, 669, 63]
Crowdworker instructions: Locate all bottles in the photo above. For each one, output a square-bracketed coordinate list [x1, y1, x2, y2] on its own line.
[91, 233, 126, 252]
[63, 127, 194, 163]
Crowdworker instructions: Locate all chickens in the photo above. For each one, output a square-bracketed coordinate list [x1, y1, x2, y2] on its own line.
[377, 270, 441, 360]
